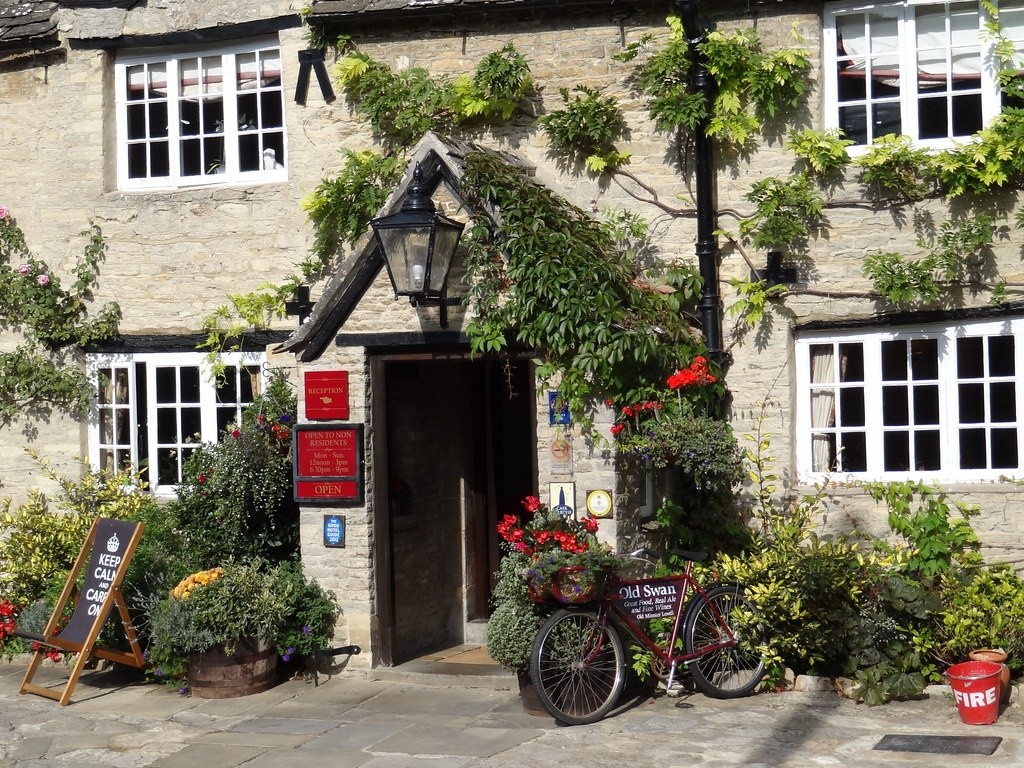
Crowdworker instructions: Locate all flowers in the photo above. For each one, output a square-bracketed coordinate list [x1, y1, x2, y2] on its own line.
[496, 496, 599, 555]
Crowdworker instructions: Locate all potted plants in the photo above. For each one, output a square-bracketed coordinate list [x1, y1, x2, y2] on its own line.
[486, 553, 562, 716]
[135, 564, 336, 699]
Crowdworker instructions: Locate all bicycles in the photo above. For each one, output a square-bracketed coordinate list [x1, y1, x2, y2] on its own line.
[525, 545, 770, 725]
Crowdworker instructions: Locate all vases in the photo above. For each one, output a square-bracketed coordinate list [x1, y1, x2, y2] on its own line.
[532, 551, 622, 605]
[969, 651, 1011, 699]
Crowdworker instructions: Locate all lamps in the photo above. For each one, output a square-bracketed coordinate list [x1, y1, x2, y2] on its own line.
[367, 160, 466, 328]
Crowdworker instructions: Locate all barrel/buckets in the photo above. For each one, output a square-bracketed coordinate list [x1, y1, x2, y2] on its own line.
[947, 661, 1003, 725]
